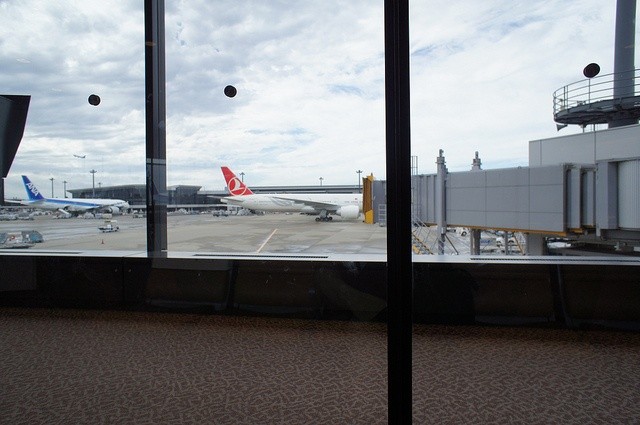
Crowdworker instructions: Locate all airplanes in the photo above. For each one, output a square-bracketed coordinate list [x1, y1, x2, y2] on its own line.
[73, 153, 86, 158]
[1, 176, 131, 218]
[220, 166, 364, 221]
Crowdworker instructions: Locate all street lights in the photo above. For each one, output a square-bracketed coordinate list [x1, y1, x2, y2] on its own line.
[90, 168, 96, 197]
[355, 169, 363, 193]
[63, 180, 68, 198]
[49, 178, 55, 198]
[318, 177, 324, 185]
[239, 171, 245, 182]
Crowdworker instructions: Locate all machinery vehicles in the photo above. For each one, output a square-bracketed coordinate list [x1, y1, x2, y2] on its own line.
[213, 210, 228, 217]
[98, 224, 120, 232]
